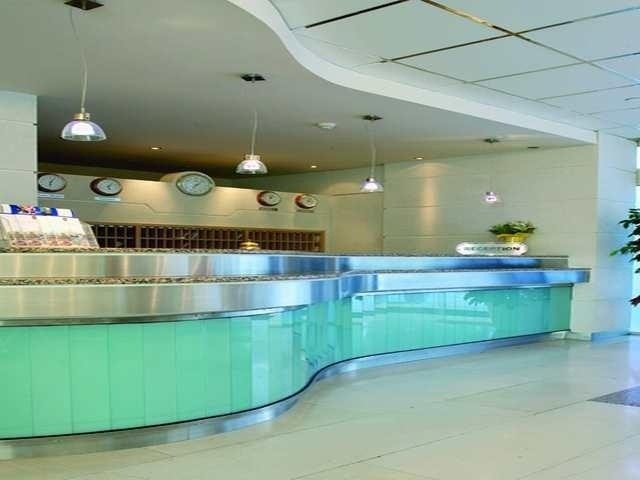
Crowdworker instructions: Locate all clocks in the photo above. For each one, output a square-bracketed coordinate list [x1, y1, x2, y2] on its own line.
[89, 177, 122, 197]
[177, 173, 213, 196]
[258, 191, 281, 206]
[294, 194, 319, 209]
[37, 173, 67, 193]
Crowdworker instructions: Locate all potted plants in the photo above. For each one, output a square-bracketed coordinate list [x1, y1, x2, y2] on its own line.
[488, 221, 536, 243]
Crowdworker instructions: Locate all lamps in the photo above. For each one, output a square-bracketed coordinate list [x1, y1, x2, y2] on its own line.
[235, 74, 269, 175]
[481, 138, 502, 204]
[60, 0, 108, 142]
[361, 116, 384, 193]
